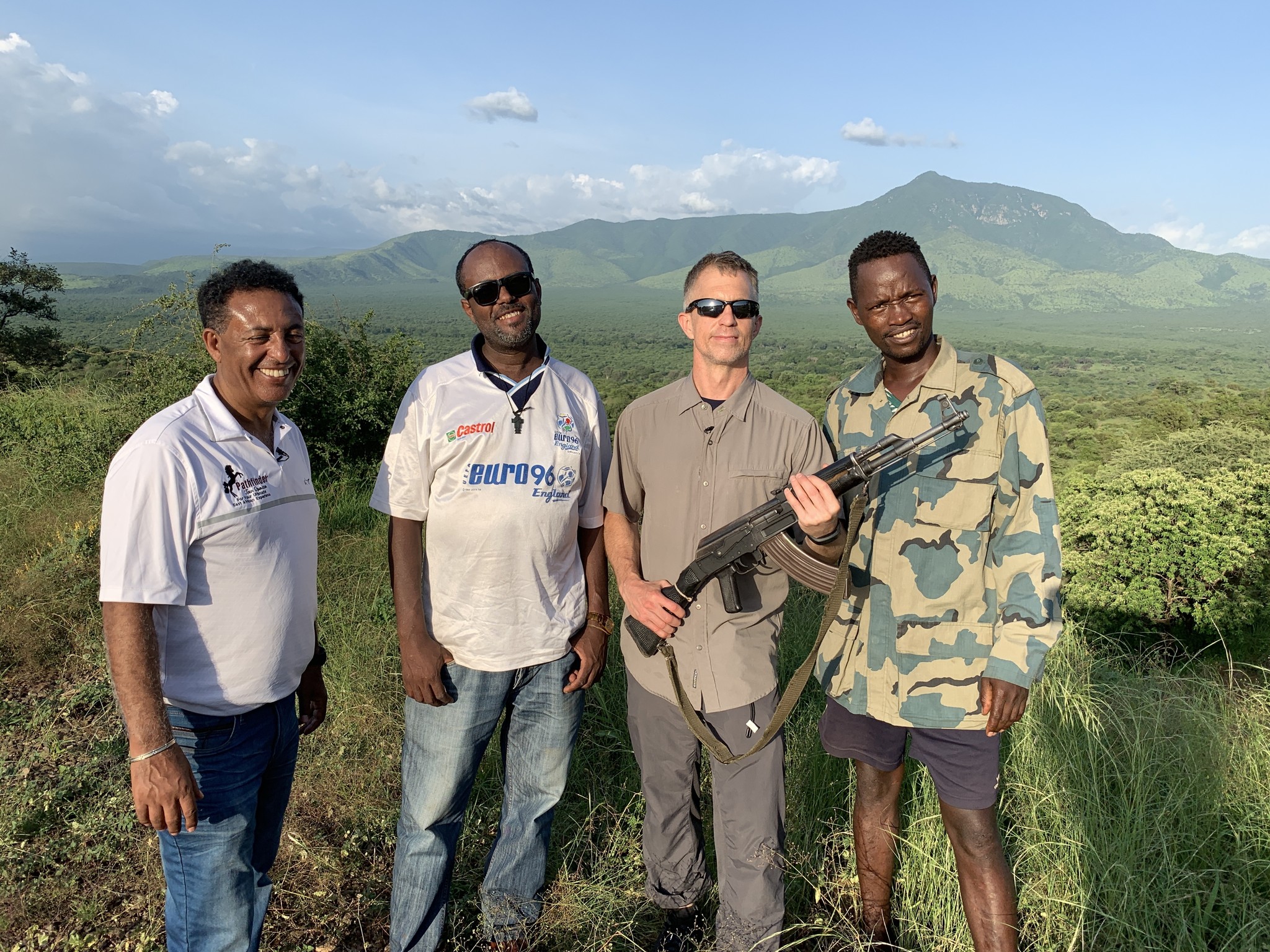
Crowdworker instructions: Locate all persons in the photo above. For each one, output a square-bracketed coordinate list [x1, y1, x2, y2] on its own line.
[598, 249, 844, 952]
[97, 259, 330, 951]
[818, 229, 1067, 952]
[370, 236, 608, 952]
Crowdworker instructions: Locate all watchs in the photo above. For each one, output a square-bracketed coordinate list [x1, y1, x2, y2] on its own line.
[587, 612, 614, 636]
[807, 519, 842, 545]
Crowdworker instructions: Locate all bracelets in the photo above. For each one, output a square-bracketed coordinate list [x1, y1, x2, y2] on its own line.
[129, 736, 174, 763]
[312, 644, 327, 667]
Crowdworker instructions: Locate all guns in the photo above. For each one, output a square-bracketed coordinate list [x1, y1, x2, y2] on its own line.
[624, 393, 970, 658]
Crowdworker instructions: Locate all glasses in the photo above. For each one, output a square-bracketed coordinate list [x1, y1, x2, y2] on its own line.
[685, 298, 760, 319]
[464, 271, 536, 307]
